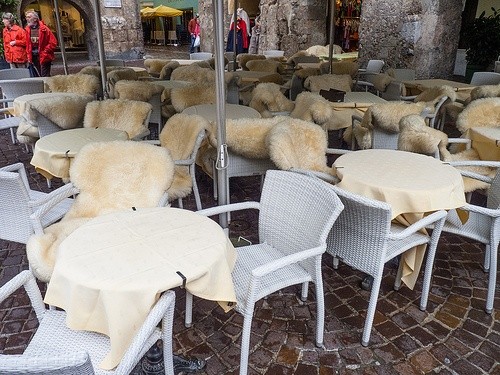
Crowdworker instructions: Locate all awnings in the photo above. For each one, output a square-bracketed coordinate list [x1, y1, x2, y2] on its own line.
[140, 4, 183, 17]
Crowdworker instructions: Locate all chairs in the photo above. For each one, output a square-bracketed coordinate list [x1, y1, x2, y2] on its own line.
[0, 163, 74, 284]
[0, 267, 175, 375]
[394, 167, 500, 313]
[0, 42, 500, 210]
[183, 169, 346, 375]
[27, 140, 174, 310]
[299, 171, 448, 350]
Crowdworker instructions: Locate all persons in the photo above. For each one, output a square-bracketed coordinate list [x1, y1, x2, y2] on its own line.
[24, 10, 57, 78]
[2, 12, 27, 69]
[188, 13, 199, 53]
[193, 16, 201, 53]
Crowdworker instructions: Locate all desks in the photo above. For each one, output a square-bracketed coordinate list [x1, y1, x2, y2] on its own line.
[56, 206, 238, 336]
